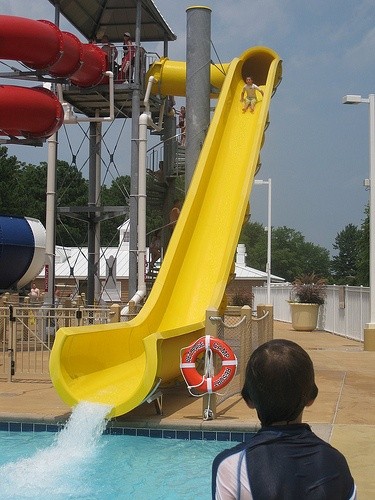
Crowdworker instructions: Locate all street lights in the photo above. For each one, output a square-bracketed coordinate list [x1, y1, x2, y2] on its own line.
[341, 93, 375, 352]
[254, 178, 272, 306]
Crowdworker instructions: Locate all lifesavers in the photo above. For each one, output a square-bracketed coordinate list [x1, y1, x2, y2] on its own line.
[181, 336, 237, 392]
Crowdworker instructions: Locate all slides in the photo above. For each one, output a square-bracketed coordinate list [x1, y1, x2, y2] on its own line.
[0, 13, 280, 419]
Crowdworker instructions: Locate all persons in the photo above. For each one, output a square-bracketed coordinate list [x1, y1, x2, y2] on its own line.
[240, 76, 264, 113]
[122, 32, 130, 60]
[31, 282, 41, 300]
[173, 105, 186, 149]
[210, 339, 359, 500]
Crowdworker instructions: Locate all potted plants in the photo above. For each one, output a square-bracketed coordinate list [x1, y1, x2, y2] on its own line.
[288, 272, 328, 331]
